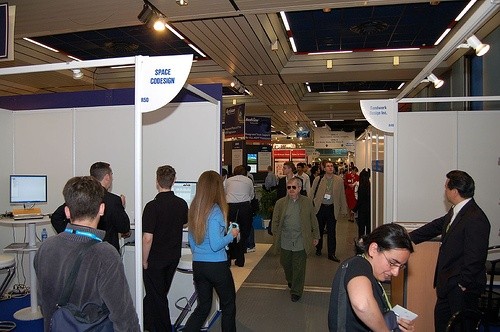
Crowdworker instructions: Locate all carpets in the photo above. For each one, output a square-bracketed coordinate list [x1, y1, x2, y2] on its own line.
[230, 243, 273, 291]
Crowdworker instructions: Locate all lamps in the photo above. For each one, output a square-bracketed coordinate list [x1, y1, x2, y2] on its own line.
[137, 3, 166, 32]
[230, 79, 238, 86]
[428, 72, 444, 89]
[71, 69, 85, 80]
[466, 34, 491, 57]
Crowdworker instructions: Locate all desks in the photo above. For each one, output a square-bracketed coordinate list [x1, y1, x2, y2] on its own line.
[0, 214, 50, 322]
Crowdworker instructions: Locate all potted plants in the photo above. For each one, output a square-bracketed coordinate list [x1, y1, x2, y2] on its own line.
[255, 187, 277, 229]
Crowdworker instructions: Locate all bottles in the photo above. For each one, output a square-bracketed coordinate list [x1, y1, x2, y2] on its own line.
[42, 228, 48, 242]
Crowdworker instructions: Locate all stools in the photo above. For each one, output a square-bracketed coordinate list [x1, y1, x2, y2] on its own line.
[0, 255, 16, 332]
[478, 245, 500, 325]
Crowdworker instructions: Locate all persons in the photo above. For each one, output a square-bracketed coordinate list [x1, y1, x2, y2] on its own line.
[51, 161, 131, 255]
[311, 161, 347, 262]
[34, 177, 141, 332]
[328, 224, 417, 332]
[272, 178, 320, 302]
[334, 162, 358, 222]
[142, 166, 189, 332]
[265, 166, 276, 190]
[223, 166, 255, 266]
[187, 170, 241, 332]
[409, 170, 491, 332]
[276, 161, 326, 197]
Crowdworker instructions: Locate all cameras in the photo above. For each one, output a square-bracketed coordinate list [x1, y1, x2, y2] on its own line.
[324, 194, 331, 199]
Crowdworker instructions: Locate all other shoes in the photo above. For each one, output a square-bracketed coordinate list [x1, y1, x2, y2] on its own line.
[316, 248, 321, 256]
[328, 256, 340, 262]
[234, 259, 244, 267]
[288, 282, 292, 288]
[247, 246, 256, 252]
[291, 294, 300, 302]
[348, 219, 354, 222]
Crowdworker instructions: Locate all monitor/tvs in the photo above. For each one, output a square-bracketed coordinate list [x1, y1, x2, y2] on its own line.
[9, 175, 48, 204]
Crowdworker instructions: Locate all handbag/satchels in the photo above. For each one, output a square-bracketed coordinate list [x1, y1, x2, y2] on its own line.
[49, 239, 114, 332]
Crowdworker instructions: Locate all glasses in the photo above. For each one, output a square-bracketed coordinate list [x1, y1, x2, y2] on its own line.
[287, 186, 299, 189]
[379, 246, 406, 270]
